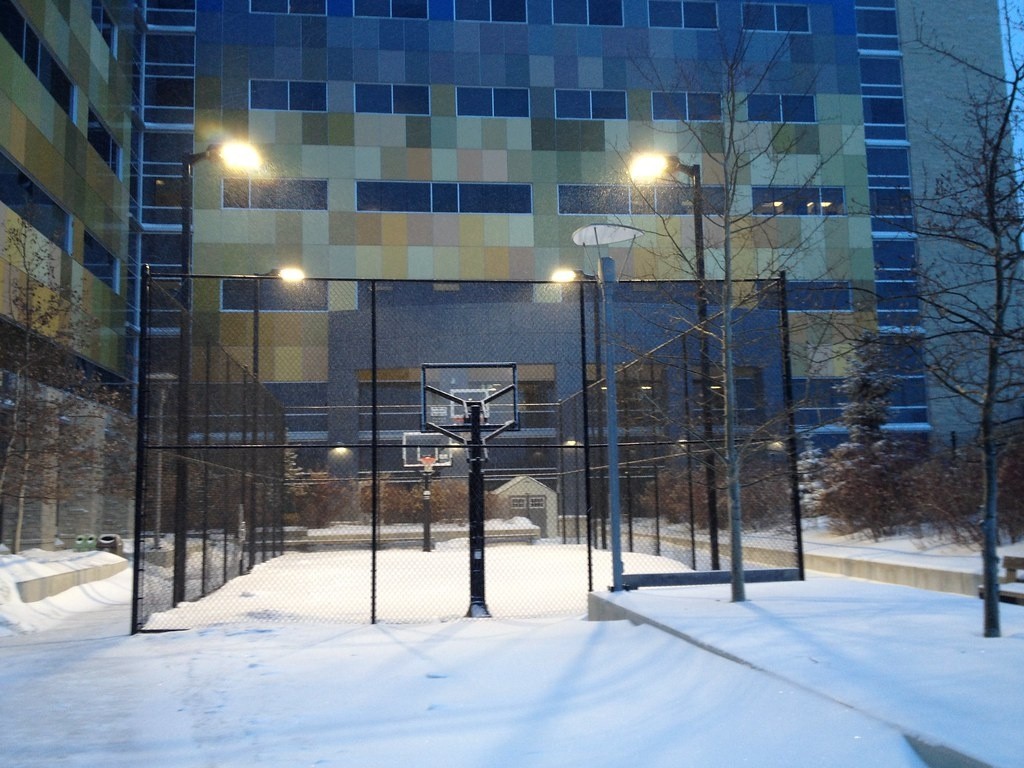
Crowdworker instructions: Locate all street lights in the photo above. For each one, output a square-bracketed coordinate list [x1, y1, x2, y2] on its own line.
[631, 152, 720, 570]
[551, 269, 608, 549]
[171, 140, 261, 610]
[248, 267, 304, 570]
